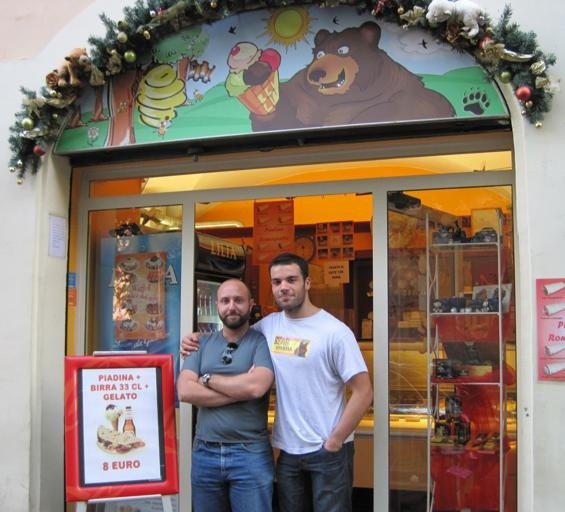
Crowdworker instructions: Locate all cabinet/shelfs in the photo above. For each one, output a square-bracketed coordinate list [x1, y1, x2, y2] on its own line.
[267, 212, 516, 511]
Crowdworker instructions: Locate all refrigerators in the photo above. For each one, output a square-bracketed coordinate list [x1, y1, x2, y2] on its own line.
[194, 231, 246, 334]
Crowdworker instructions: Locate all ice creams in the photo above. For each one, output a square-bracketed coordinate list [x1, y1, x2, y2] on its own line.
[104, 405, 123, 431]
[224, 42, 281, 118]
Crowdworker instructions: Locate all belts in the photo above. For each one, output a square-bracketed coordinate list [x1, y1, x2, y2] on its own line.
[198, 440, 233, 446]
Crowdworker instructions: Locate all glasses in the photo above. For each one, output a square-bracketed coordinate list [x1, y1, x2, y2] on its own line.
[221, 342, 238, 365]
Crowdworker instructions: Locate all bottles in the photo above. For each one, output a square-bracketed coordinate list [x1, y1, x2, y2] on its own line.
[123, 406, 135, 437]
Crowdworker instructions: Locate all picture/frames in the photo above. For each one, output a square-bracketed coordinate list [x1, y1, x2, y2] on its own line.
[64, 353, 180, 503]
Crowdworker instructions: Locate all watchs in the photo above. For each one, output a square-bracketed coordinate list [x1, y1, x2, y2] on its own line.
[202, 373, 212, 389]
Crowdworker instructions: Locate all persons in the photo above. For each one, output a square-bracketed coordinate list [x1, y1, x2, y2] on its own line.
[174, 276, 276, 511]
[179, 252, 373, 511]
